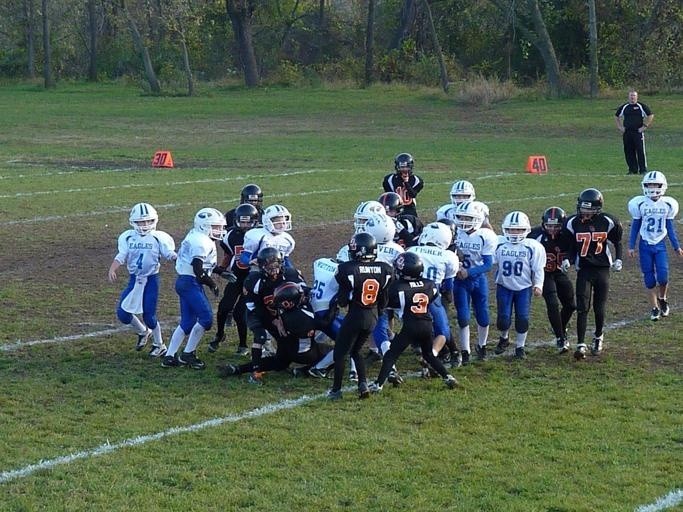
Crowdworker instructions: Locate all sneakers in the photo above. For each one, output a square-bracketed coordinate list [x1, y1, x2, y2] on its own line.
[292, 350, 403, 398]
[412, 335, 526, 390]
[592, 331, 603, 355]
[657, 296, 670, 317]
[557, 333, 570, 354]
[651, 306, 661, 321]
[574, 346, 586, 358]
[626, 172, 637, 175]
[640, 171, 648, 175]
[137, 313, 277, 385]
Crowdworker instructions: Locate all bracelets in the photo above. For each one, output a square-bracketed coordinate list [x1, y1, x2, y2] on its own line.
[643, 123, 648, 128]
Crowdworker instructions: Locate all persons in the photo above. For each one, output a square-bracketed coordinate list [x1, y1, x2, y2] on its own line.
[211, 154, 582, 401]
[614, 90, 654, 176]
[107, 202, 177, 359]
[563, 189, 623, 360]
[627, 172, 682, 321]
[162, 208, 237, 370]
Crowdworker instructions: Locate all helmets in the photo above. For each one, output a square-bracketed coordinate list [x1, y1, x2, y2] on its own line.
[577, 188, 603, 216]
[541, 206, 567, 241]
[128, 203, 158, 236]
[502, 210, 531, 244]
[336, 152, 490, 282]
[194, 184, 304, 311]
[642, 171, 668, 198]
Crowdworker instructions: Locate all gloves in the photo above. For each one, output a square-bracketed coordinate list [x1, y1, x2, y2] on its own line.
[560, 259, 570, 274]
[611, 259, 622, 272]
